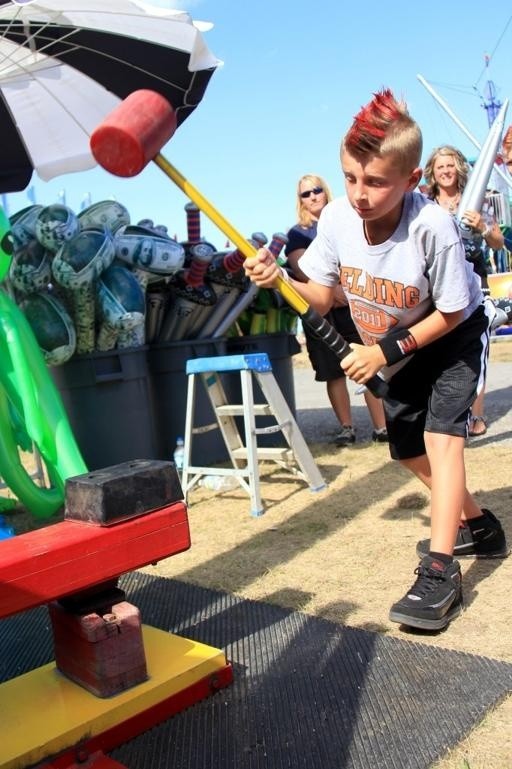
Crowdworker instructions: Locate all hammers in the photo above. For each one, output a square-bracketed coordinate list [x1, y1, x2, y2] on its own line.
[90, 89, 390, 398]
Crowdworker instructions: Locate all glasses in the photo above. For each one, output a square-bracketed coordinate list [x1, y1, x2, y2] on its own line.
[299, 187, 323, 198]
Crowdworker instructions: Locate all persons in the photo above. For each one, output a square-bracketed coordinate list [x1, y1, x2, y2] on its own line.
[242, 84, 511, 631]
[285, 173, 390, 443]
[422, 145, 503, 437]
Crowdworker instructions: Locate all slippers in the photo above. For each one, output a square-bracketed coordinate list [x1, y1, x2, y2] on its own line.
[467, 415, 488, 437]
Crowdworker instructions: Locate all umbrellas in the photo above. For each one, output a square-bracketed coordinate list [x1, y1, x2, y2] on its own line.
[0, 0, 223, 194]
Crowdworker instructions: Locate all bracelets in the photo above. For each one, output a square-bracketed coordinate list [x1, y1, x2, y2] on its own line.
[379, 329, 418, 365]
[481, 223, 490, 239]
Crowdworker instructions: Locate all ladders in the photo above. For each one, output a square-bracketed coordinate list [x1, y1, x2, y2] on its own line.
[181, 354, 327, 516]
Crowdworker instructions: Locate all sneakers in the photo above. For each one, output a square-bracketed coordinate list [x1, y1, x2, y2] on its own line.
[328, 424, 357, 448]
[415, 508, 510, 558]
[389, 556, 463, 630]
[371, 426, 389, 441]
